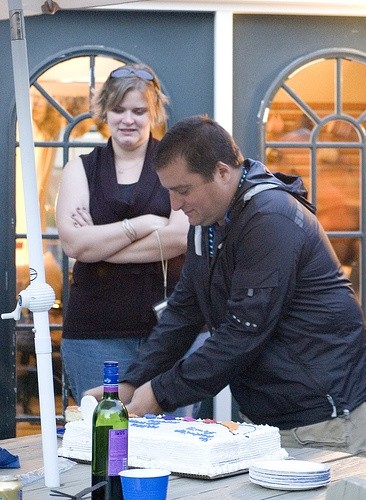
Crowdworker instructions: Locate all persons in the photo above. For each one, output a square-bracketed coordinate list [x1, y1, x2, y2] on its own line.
[81, 114, 366, 459]
[54, 63, 212, 418]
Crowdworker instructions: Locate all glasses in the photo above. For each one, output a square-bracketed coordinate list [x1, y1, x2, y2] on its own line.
[110, 68, 154, 83]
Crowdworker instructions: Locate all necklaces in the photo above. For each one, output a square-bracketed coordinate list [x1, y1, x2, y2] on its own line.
[115, 158, 144, 174]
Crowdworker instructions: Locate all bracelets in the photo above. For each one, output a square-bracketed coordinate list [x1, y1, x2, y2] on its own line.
[121, 219, 137, 242]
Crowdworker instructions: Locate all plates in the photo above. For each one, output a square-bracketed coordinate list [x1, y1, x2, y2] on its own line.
[248, 460, 331, 492]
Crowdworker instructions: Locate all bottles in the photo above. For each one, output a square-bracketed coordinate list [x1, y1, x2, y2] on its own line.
[90, 360, 129, 500]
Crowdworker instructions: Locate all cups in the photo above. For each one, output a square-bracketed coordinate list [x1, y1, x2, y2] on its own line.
[118, 468, 171, 500]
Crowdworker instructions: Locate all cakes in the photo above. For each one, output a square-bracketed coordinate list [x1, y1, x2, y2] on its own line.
[61, 395, 287, 478]
